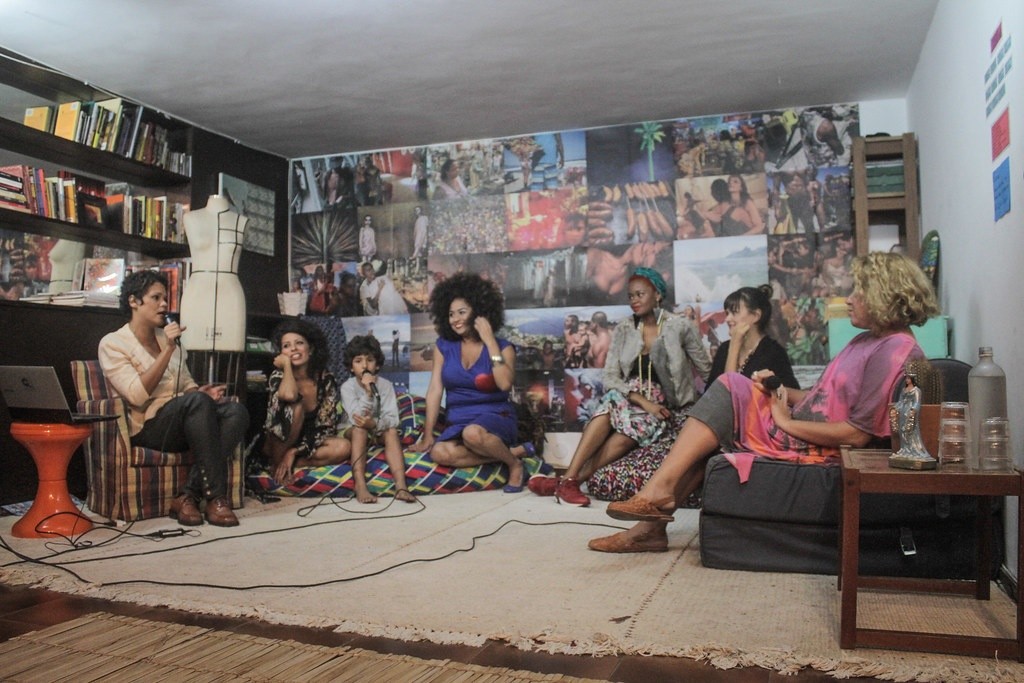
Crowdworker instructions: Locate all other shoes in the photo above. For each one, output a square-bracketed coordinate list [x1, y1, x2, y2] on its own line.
[502, 465, 526, 492]
[523, 441, 535, 456]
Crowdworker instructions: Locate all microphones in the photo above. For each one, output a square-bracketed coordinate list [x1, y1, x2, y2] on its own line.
[761, 376, 781, 389]
[165, 314, 181, 347]
[361, 370, 380, 401]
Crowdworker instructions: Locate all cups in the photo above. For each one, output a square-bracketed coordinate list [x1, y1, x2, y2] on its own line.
[938, 400, 969, 474]
[979, 416, 1011, 473]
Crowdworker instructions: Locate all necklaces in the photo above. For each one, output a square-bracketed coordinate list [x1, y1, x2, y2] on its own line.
[737, 349, 755, 374]
[638, 306, 664, 401]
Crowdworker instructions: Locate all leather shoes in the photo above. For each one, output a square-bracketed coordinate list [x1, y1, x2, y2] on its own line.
[169, 493, 202, 525]
[204, 496, 239, 527]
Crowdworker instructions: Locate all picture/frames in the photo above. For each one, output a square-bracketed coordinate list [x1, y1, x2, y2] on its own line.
[76, 191, 108, 229]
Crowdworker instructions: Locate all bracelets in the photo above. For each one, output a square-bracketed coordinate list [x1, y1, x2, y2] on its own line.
[490, 356, 505, 367]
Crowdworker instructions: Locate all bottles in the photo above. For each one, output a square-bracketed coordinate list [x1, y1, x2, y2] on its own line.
[968, 346, 1006, 470]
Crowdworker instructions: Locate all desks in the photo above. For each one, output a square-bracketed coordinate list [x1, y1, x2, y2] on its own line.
[10, 422, 93, 538]
[838, 446, 1024, 658]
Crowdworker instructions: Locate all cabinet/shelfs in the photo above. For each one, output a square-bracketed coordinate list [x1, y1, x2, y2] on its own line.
[220, 349, 281, 399]
[0, 115, 192, 319]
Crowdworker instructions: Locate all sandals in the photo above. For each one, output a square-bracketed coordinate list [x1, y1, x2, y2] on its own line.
[588, 530, 668, 553]
[607, 492, 676, 522]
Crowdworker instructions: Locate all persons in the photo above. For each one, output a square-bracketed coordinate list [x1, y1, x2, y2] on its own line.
[704, 284, 801, 394]
[336, 335, 417, 503]
[97, 269, 251, 527]
[528, 266, 713, 508]
[888, 373, 935, 462]
[521, 155, 534, 188]
[678, 105, 858, 299]
[292, 155, 426, 315]
[392, 330, 401, 368]
[179, 194, 251, 351]
[587, 252, 942, 553]
[431, 142, 504, 199]
[252, 319, 352, 484]
[541, 311, 613, 371]
[587, 241, 673, 308]
[407, 270, 537, 493]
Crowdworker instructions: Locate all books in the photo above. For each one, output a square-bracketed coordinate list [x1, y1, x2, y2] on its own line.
[0, 95, 194, 313]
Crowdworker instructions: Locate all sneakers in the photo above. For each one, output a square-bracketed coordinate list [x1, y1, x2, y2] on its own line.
[528, 474, 563, 497]
[554, 477, 590, 507]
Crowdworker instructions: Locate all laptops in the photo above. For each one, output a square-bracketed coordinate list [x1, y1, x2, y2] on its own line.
[0, 365, 121, 425]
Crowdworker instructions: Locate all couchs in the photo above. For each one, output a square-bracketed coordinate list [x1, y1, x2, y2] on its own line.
[699, 358, 1002, 576]
[71, 359, 244, 522]
